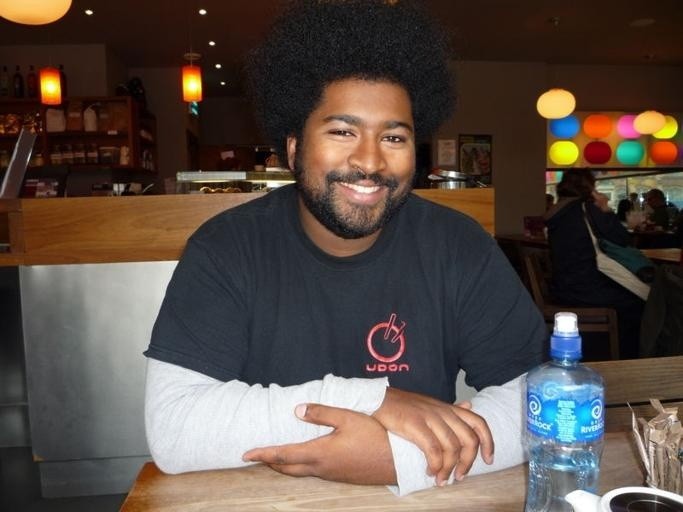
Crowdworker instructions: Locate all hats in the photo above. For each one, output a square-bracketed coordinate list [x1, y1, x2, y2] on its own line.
[642, 189, 665, 210]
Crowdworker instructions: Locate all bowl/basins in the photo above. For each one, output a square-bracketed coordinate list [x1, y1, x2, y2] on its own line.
[253, 165, 263, 171]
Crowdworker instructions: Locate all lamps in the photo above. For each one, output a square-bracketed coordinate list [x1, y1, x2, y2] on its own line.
[536, 87, 679, 167]
[181, 29, 204, 117]
[37, 53, 62, 105]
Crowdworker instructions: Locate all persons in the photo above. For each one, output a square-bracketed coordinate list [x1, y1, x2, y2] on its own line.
[142, 1, 578, 496]
[543, 167, 683, 358]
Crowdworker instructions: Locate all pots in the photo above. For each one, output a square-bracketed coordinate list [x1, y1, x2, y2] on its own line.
[427, 167, 486, 188]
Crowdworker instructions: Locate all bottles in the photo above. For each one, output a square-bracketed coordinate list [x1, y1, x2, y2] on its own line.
[0, 63, 67, 98]
[524, 309, 606, 511]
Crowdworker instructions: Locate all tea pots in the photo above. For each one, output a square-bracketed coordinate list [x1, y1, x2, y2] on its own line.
[625, 210, 645, 224]
[562, 485, 683, 511]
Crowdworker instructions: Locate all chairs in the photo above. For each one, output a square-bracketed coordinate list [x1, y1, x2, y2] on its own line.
[579, 356, 683, 432]
[525, 255, 620, 360]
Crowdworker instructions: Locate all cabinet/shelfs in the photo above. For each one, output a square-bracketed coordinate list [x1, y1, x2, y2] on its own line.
[0, 96, 158, 199]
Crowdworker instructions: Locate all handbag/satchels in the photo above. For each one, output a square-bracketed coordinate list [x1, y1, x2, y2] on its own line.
[599, 238, 655, 283]
[596, 251, 651, 301]
